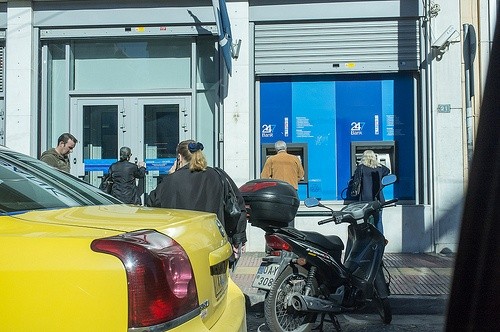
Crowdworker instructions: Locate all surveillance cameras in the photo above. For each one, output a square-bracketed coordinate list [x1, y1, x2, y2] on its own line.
[431, 25, 456, 51]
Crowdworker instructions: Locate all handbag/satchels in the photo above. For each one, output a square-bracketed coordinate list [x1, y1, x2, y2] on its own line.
[211, 166, 242, 238]
[350, 165, 362, 197]
[99, 167, 114, 194]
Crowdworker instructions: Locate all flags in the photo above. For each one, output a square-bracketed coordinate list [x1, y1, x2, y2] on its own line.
[210, 0, 233, 78]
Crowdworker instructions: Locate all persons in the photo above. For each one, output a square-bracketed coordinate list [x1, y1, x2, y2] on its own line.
[260, 140, 304, 228]
[146, 139, 247, 271]
[40, 133, 77, 174]
[350, 150, 390, 235]
[98, 146, 147, 206]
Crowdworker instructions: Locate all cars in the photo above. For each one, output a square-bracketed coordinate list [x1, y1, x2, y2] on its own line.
[0, 144, 247, 331]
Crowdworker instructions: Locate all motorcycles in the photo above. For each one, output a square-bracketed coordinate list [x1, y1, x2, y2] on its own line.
[237, 173, 399, 332]
